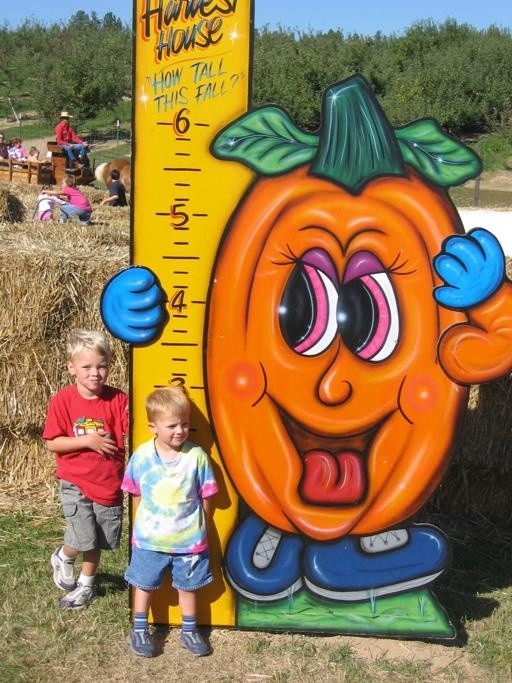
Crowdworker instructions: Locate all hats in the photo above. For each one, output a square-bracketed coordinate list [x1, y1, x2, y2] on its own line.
[60, 112, 72, 117]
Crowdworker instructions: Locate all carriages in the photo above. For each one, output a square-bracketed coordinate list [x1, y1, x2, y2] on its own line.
[0, 138, 131, 202]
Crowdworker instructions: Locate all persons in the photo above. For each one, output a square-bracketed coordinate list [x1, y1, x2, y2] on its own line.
[117, 386, 221, 658]
[38, 174, 93, 225]
[55, 112, 93, 168]
[0, 133, 40, 169]
[40, 328, 129, 610]
[100, 170, 128, 207]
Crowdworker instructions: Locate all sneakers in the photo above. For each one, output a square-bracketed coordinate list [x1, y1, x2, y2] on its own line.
[127, 632, 154, 657]
[181, 630, 210, 656]
[51, 547, 97, 609]
